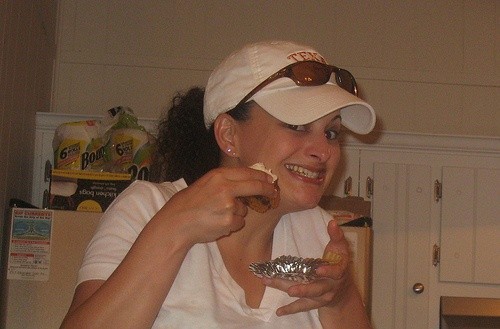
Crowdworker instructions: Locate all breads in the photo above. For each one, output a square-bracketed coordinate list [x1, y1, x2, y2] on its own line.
[241, 163, 281, 213]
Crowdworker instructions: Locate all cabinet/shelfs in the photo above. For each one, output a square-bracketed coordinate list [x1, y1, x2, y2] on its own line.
[325, 143, 499, 329]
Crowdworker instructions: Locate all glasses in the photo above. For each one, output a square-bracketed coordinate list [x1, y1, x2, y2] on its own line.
[230, 61, 357, 116]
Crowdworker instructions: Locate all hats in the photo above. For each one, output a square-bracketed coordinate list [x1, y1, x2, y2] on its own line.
[203, 41, 375, 135]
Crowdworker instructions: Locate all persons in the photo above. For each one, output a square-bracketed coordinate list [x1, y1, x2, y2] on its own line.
[59, 39, 376, 329]
